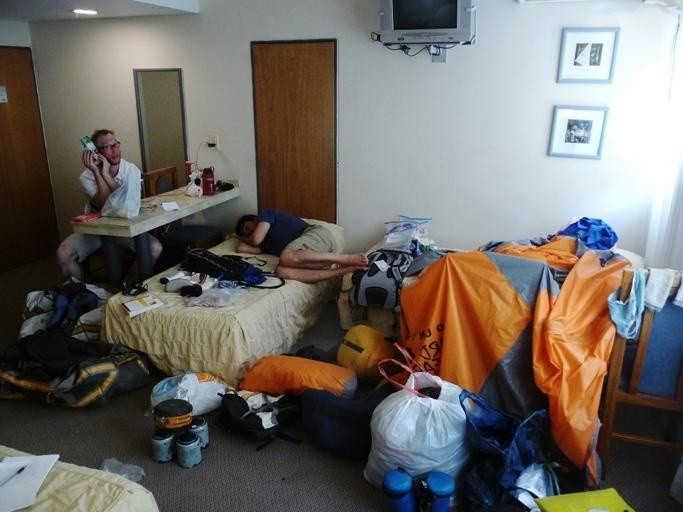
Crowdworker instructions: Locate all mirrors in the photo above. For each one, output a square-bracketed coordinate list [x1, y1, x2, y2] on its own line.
[133, 67, 189, 197]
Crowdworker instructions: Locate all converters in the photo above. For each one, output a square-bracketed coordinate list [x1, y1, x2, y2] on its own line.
[207, 139, 216, 147]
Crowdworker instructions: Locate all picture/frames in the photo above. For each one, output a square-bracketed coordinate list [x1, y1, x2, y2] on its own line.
[547, 104, 610, 161]
[556, 27, 621, 84]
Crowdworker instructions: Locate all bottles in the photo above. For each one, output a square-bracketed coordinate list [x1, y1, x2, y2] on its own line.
[382, 468, 456, 512]
[184, 162, 195, 186]
[202, 168, 214, 195]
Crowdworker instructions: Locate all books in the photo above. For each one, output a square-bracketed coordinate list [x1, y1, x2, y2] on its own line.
[119, 293, 164, 320]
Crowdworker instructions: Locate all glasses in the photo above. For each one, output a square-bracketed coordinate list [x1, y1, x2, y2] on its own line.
[99, 142, 120, 150]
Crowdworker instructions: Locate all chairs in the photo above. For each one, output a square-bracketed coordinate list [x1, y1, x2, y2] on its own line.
[598, 269, 683, 480]
[144, 165, 223, 258]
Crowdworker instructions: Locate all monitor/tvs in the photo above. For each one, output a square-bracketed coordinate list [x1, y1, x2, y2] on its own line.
[378, 0, 471, 44]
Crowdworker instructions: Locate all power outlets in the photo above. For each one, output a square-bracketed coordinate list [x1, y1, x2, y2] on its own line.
[431, 43, 446, 64]
[207, 135, 219, 153]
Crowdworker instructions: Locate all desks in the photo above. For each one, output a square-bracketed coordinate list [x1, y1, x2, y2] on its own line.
[70, 187, 240, 285]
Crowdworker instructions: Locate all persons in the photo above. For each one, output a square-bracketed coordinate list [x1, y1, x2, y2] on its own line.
[230, 208, 370, 285]
[53, 129, 162, 288]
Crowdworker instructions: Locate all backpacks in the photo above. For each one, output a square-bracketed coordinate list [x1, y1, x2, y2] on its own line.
[348, 249, 412, 329]
[0, 350, 156, 408]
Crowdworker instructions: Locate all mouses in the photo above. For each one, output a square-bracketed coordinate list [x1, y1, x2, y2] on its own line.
[129, 287, 147, 297]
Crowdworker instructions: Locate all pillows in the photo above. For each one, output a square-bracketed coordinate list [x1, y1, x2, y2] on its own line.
[237, 354, 358, 399]
[350, 251, 413, 311]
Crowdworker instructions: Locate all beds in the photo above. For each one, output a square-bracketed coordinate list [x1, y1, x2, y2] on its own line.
[0, 444, 159, 512]
[337, 244, 646, 348]
[100, 221, 344, 391]
